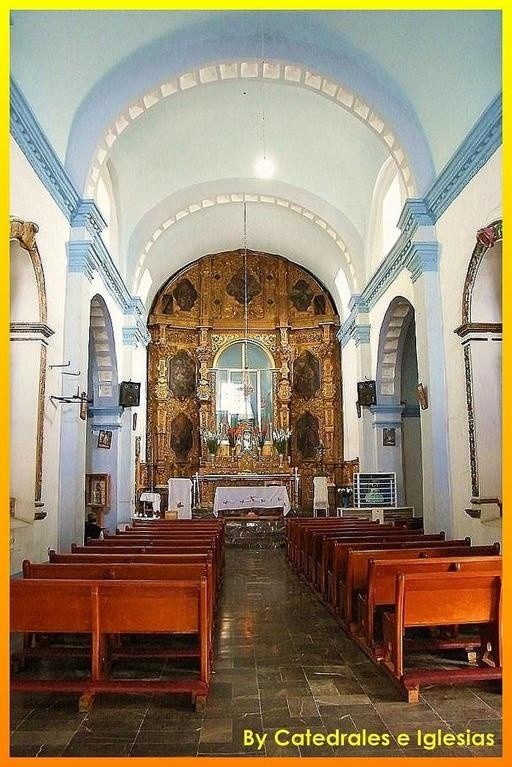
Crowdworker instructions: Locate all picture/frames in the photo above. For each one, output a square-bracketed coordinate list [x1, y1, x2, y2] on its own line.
[383, 428, 395, 447]
[98, 429, 112, 449]
[416, 383, 428, 410]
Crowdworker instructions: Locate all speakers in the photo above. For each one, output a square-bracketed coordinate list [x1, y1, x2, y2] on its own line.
[357, 379, 375, 405]
[120, 381, 141, 406]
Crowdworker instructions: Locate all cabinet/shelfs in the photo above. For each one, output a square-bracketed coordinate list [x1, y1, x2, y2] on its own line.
[352, 472, 398, 508]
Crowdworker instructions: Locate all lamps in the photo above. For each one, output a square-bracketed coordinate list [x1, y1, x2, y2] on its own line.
[236, 201, 256, 396]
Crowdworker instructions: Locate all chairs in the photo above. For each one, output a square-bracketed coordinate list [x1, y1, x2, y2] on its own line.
[313, 476, 329, 518]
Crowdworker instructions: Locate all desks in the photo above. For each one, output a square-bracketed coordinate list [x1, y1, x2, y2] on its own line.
[140, 492, 161, 518]
[212, 486, 291, 518]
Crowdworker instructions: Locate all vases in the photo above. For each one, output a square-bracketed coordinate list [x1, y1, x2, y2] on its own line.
[257, 447, 262, 460]
[211, 454, 215, 467]
[278, 454, 284, 468]
[231, 447, 235, 463]
[343, 497, 349, 508]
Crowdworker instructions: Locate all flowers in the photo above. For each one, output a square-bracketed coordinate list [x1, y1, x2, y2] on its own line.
[252, 426, 268, 445]
[337, 487, 353, 497]
[272, 427, 293, 454]
[225, 421, 247, 446]
[203, 431, 224, 454]
